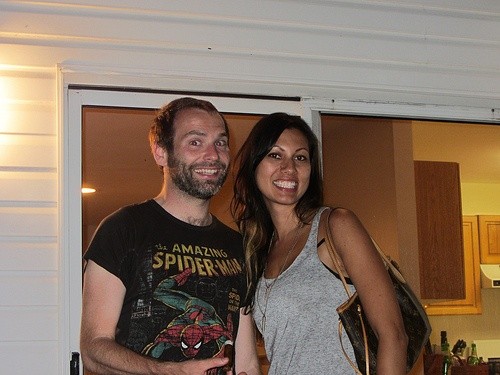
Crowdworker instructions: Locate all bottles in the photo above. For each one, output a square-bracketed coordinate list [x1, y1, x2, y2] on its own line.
[478, 356, 485, 365]
[71, 352, 80, 375]
[467, 343, 479, 365]
[438, 330, 452, 375]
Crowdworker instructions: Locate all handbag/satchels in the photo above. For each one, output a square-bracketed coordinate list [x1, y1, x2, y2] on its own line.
[323, 207, 432, 375]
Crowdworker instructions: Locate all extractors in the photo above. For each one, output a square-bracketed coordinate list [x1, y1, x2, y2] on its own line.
[480, 263, 500, 289]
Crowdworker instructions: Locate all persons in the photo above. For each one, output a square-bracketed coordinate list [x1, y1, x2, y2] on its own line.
[235, 112, 408, 375]
[80, 97, 261, 375]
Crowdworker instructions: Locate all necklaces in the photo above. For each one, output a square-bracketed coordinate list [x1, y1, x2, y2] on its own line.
[257, 223, 304, 333]
